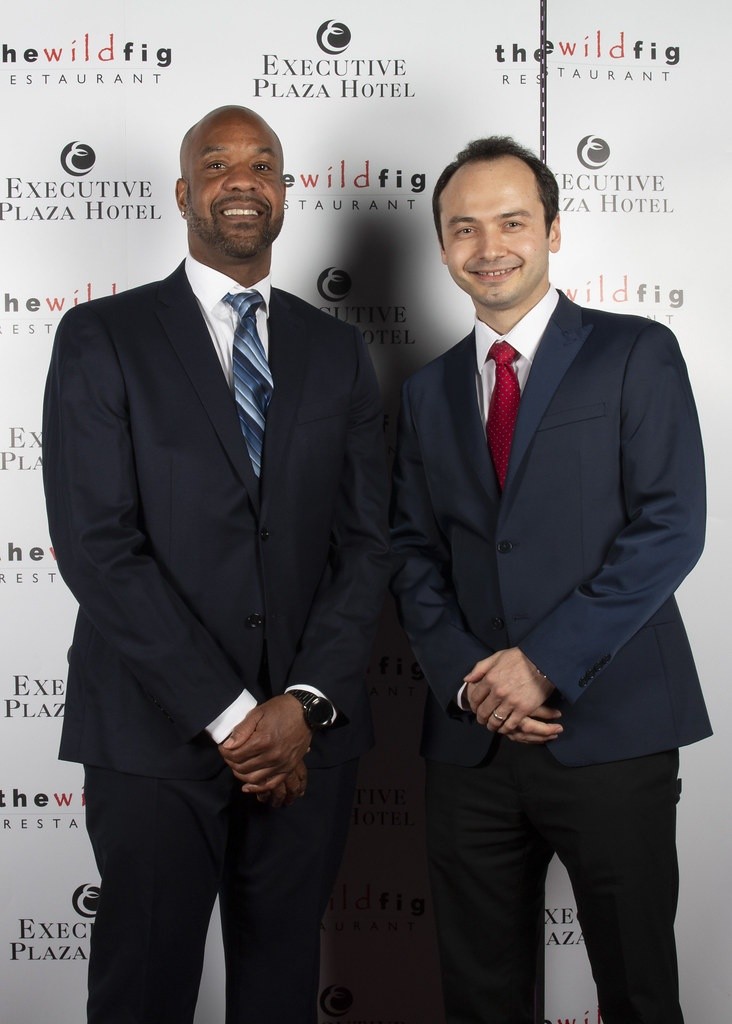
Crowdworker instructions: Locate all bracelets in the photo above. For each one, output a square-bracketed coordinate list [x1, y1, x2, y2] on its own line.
[537, 669, 546, 679]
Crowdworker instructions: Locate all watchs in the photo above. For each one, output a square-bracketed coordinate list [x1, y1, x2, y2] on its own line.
[287, 689, 333, 731]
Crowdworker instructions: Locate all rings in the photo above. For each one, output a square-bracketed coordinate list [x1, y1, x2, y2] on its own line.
[493, 711, 502, 720]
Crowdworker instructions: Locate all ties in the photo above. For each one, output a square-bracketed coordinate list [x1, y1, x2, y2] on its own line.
[222, 290, 274, 478]
[485, 341, 521, 493]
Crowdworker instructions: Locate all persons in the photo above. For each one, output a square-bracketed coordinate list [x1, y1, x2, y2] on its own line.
[390, 136, 716, 1024]
[41, 104, 390, 1024]
